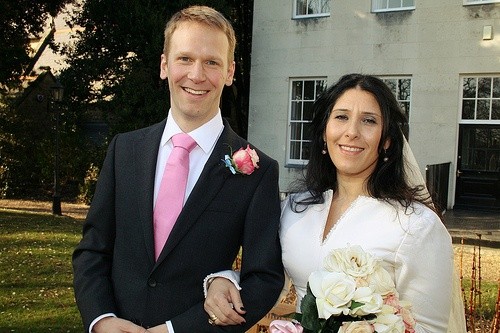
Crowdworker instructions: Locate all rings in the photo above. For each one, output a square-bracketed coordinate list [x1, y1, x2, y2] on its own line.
[208, 316, 218, 325]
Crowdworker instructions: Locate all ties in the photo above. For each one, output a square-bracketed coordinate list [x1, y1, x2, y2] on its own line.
[153, 133, 197, 262]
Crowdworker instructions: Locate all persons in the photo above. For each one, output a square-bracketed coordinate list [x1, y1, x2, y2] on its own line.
[72, 6, 284, 333]
[202, 74, 454, 333]
